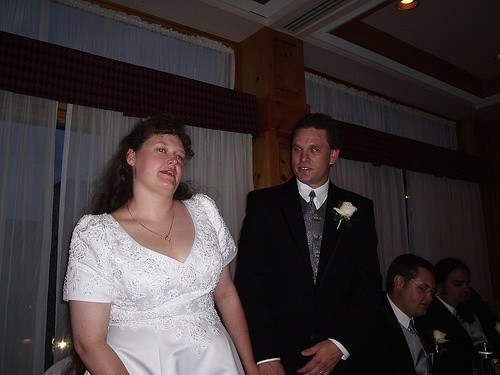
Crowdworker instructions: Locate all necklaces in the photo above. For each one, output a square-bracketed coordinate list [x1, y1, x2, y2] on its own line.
[126, 199, 174, 245]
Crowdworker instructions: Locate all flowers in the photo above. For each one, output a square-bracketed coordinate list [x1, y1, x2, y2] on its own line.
[433, 330, 449, 345]
[331, 200, 359, 228]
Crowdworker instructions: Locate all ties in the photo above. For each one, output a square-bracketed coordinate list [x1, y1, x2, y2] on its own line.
[408, 320, 416, 334]
[308, 191, 316, 210]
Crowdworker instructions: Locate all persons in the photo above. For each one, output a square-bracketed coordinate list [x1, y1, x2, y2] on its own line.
[367, 253, 476, 375]
[233, 112, 385, 375]
[428, 257, 500, 375]
[63, 112, 260, 375]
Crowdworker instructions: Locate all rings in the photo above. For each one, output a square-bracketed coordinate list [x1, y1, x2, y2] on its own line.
[319, 371, 323, 375]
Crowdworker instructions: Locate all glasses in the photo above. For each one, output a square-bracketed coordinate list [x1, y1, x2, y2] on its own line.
[416, 283, 439, 298]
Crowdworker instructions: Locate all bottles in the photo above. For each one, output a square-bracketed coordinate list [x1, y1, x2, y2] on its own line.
[481, 342, 493, 375]
